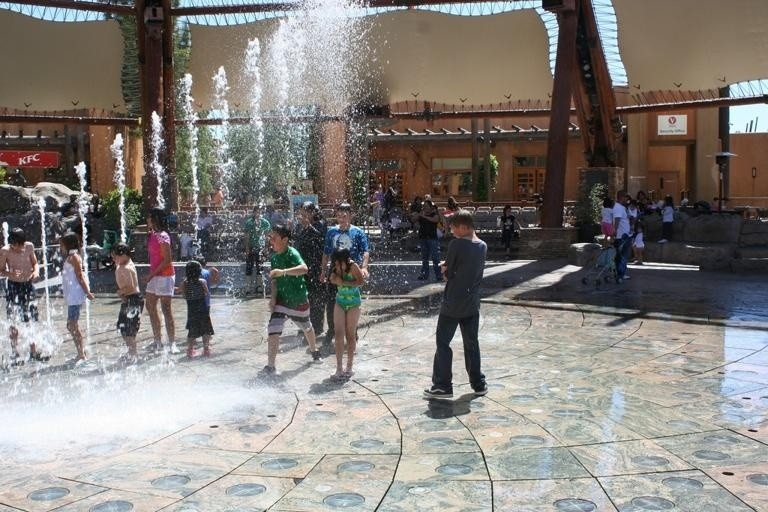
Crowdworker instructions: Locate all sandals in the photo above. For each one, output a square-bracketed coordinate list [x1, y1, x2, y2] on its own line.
[330, 369, 356, 382]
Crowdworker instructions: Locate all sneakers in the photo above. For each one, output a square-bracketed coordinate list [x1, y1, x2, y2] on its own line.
[309, 347, 325, 364]
[255, 365, 277, 379]
[422, 374, 487, 399]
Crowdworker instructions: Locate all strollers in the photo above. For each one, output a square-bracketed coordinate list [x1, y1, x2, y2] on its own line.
[582, 245, 623, 288]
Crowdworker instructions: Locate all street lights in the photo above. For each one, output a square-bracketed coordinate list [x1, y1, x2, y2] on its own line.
[706, 151, 739, 213]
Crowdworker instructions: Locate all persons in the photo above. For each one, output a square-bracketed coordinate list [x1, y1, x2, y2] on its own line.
[295, 201, 328, 336]
[256, 224, 325, 377]
[142, 207, 180, 355]
[0, 178, 765, 286]
[189, 256, 218, 336]
[319, 203, 369, 343]
[422, 209, 489, 399]
[109, 242, 145, 365]
[180, 260, 215, 359]
[329, 247, 365, 380]
[0, 226, 51, 366]
[58, 231, 95, 362]
